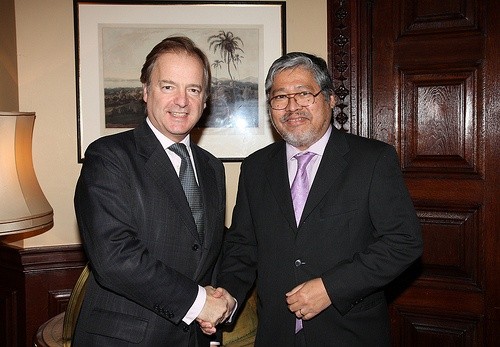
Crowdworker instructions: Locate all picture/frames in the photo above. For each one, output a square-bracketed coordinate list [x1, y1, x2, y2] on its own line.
[73, 0, 287, 164]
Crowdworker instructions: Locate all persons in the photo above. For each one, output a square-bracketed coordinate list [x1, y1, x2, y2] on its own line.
[196, 51, 424, 347]
[71, 36, 229, 347]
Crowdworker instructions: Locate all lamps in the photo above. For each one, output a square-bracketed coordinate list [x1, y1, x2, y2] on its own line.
[0, 111, 53, 236]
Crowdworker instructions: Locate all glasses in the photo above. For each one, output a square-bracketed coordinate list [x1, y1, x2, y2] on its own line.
[267, 88, 324, 110]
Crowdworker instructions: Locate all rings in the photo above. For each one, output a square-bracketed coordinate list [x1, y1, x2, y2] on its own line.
[300, 310, 304, 316]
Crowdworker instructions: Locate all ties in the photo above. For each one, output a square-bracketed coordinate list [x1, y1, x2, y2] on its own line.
[166, 143, 204, 245]
[290, 152, 318, 334]
